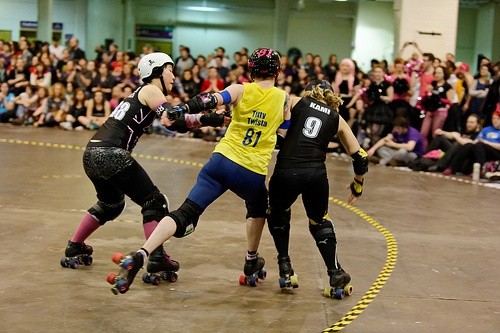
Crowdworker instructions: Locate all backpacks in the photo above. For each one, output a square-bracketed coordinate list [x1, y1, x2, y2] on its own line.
[411, 157, 445, 173]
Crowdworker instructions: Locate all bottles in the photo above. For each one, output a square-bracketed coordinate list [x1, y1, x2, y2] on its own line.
[24, 114, 28, 126]
[472, 163, 480, 181]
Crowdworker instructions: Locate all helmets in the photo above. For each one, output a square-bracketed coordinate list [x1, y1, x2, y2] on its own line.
[248, 48, 283, 78]
[305, 79, 335, 94]
[138, 52, 175, 83]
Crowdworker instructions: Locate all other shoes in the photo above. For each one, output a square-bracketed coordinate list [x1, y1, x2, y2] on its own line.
[481, 165, 500, 179]
[443, 167, 456, 175]
[10, 114, 104, 131]
[370, 156, 406, 166]
[329, 146, 350, 159]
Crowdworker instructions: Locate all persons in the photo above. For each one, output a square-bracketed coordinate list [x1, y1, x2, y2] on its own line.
[144, 45, 339, 151]
[328, 53, 500, 181]
[266, 79, 368, 299]
[60, 52, 231, 286]
[0, 34, 161, 131]
[106, 47, 291, 295]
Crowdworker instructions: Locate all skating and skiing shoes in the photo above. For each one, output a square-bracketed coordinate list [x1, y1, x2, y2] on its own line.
[323, 264, 353, 299]
[106, 250, 145, 296]
[142, 245, 180, 286]
[60, 239, 93, 269]
[277, 256, 299, 289]
[239, 252, 267, 288]
